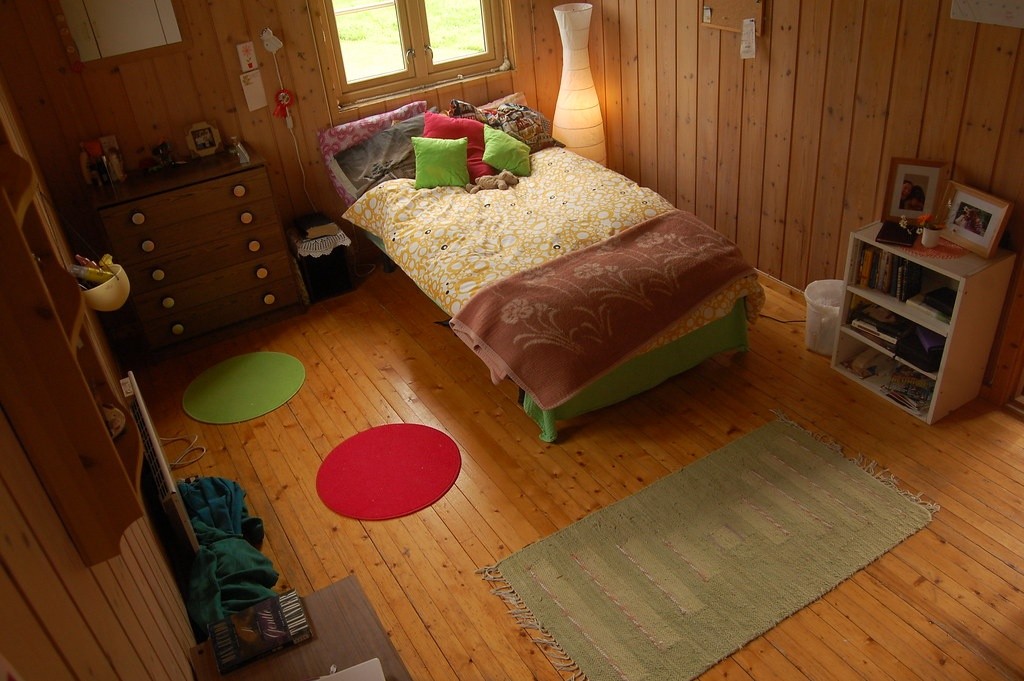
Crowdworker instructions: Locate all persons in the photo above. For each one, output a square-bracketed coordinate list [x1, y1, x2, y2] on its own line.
[956, 207, 982, 234]
[899, 180, 925, 211]
[195, 129, 214, 150]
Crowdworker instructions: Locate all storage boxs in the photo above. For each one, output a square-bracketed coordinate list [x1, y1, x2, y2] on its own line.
[287, 227, 354, 302]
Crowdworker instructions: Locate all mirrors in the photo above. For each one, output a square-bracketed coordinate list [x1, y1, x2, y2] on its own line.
[48, 0, 193, 73]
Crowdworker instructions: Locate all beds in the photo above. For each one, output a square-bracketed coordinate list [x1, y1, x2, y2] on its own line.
[317, 95, 766, 442]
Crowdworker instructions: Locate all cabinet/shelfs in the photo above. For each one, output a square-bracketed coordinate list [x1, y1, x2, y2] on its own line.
[0, 116, 146, 566]
[831, 220, 1017, 426]
[88, 141, 308, 356]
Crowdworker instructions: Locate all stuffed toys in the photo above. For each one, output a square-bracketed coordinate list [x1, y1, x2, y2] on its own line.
[466, 169, 519, 194]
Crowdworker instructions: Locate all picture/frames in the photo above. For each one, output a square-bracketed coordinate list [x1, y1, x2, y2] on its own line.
[183, 119, 225, 159]
[881, 156, 953, 228]
[931, 180, 1015, 259]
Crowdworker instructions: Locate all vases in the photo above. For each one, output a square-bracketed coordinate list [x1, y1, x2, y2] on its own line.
[921, 226, 942, 248]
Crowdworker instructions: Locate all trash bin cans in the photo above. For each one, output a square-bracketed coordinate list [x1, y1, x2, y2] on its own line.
[803, 278, 844, 358]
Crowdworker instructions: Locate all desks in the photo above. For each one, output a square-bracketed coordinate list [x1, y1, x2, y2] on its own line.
[190, 575, 414, 681]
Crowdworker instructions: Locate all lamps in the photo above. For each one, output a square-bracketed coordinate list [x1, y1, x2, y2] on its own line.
[552, 3, 606, 167]
[260, 28, 283, 54]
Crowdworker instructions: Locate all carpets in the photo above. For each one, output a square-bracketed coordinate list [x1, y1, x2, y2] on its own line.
[182, 349, 306, 424]
[475, 408, 940, 681]
[316, 424, 461, 520]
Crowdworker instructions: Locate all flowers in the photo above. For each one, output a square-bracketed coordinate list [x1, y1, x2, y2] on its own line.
[899, 199, 966, 235]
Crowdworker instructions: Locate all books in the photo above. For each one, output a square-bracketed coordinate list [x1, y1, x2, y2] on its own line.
[844, 220, 957, 416]
[292, 212, 338, 239]
[207, 588, 314, 676]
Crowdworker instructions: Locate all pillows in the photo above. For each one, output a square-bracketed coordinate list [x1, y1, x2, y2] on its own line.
[412, 136, 471, 189]
[450, 92, 566, 155]
[481, 124, 531, 177]
[422, 111, 494, 184]
